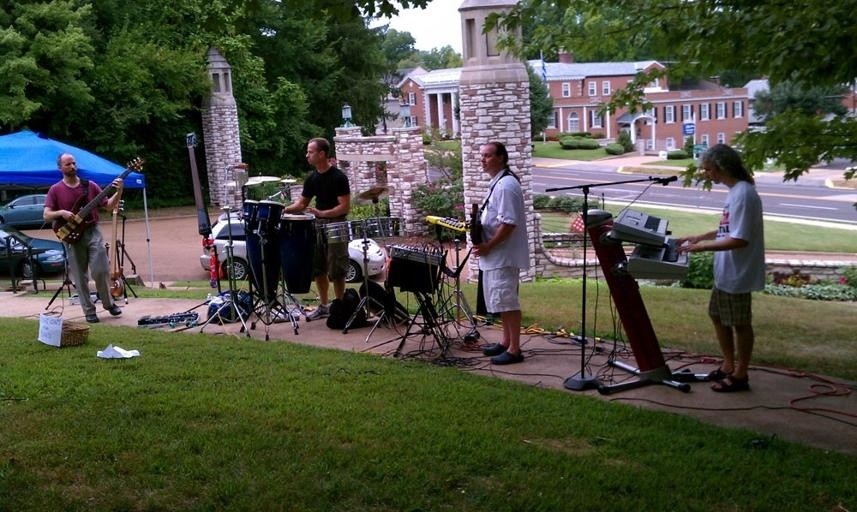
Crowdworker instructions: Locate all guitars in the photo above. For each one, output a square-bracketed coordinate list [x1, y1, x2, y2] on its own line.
[468, 202, 484, 249]
[53, 156, 147, 245]
[100, 185, 128, 298]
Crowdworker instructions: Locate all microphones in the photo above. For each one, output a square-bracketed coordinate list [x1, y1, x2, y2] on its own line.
[651, 175, 678, 187]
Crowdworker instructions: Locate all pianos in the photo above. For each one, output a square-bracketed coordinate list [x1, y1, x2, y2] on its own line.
[627, 237, 692, 280]
[612, 208, 668, 246]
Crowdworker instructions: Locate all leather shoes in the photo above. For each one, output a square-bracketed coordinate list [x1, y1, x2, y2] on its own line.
[108, 304, 122, 316]
[85, 314, 100, 324]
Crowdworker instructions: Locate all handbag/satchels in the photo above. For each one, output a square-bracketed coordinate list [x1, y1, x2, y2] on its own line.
[206, 288, 256, 325]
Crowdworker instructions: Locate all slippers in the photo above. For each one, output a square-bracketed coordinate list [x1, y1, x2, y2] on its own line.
[483, 342, 507, 356]
[491, 351, 525, 365]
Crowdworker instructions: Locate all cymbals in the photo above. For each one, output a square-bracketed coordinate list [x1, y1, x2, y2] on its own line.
[351, 184, 393, 205]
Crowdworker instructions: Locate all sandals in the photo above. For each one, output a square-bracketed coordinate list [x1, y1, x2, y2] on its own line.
[709, 367, 735, 382]
[709, 374, 749, 392]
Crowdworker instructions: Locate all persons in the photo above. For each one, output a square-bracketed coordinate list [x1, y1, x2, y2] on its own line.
[281, 137, 351, 320]
[674, 143, 766, 393]
[470, 141, 530, 365]
[42, 153, 124, 323]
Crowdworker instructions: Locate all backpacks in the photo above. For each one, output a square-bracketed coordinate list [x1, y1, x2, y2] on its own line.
[326, 288, 365, 330]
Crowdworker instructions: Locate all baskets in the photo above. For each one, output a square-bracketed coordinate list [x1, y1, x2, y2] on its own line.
[60, 320, 90, 346]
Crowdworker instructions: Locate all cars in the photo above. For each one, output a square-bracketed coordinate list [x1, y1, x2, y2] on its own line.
[200, 212, 387, 283]
[0, 194, 48, 228]
[0, 223, 69, 278]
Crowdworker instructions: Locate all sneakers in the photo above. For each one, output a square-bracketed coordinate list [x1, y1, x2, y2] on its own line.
[307, 304, 331, 320]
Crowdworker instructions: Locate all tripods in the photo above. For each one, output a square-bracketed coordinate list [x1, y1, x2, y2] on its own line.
[424, 239, 480, 340]
[342, 237, 408, 334]
[94, 215, 137, 306]
[45, 243, 76, 310]
[365, 238, 421, 345]
[240, 235, 299, 342]
[201, 245, 251, 338]
[251, 268, 311, 331]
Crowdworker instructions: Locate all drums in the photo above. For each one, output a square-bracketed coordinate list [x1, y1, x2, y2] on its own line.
[256, 200, 284, 225]
[282, 215, 317, 294]
[247, 221, 280, 294]
[369, 216, 405, 242]
[348, 220, 376, 240]
[314, 220, 350, 245]
[243, 199, 259, 222]
